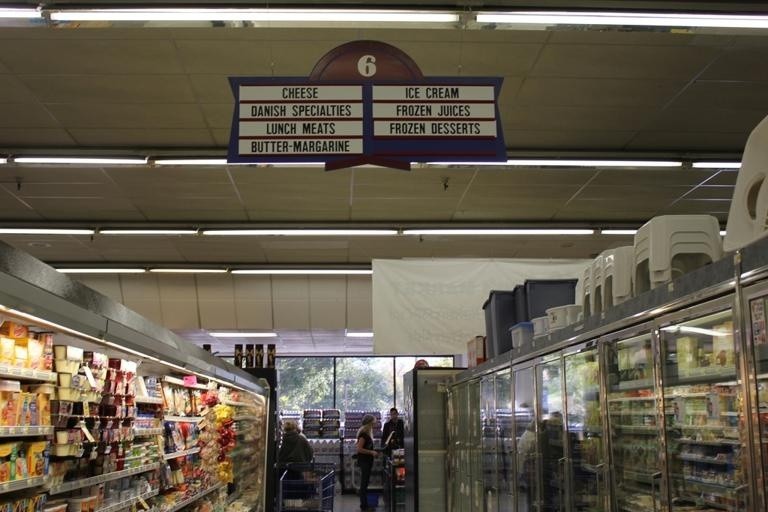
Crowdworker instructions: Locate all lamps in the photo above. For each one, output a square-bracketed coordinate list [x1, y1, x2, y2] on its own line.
[231, 264, 372, 273]
[692, 152, 743, 168]
[13, 155, 151, 166]
[150, 264, 229, 273]
[403, 223, 594, 235]
[469, 3, 766, 33]
[208, 329, 277, 337]
[2, 223, 96, 235]
[202, 223, 399, 236]
[345, 329, 374, 337]
[602, 222, 726, 236]
[54, 264, 148, 273]
[0, 1, 43, 26]
[410, 151, 685, 168]
[47, 0, 466, 29]
[99, 226, 199, 235]
[1, 156, 9, 163]
[155, 150, 387, 169]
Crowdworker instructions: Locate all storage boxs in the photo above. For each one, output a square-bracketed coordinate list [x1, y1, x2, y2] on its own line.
[482, 277, 579, 358]
[509, 322, 533, 348]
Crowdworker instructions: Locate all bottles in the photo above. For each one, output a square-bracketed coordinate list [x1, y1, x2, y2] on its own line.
[281, 409, 405, 496]
[145, 375, 162, 399]
[137, 414, 156, 428]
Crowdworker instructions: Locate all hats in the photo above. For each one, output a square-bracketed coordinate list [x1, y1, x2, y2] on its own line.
[361, 414, 373, 426]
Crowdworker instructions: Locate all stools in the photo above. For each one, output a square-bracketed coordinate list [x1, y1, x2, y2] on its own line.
[582, 246, 634, 318]
[722, 117, 767, 254]
[632, 215, 722, 297]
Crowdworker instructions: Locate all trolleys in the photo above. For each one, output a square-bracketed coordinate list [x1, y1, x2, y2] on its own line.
[276, 460, 338, 511]
[381, 452, 406, 512]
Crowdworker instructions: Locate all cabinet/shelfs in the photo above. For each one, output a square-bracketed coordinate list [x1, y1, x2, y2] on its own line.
[0, 239, 271, 512]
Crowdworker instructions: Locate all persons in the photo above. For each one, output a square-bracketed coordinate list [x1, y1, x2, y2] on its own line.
[481, 410, 581, 512]
[380, 408, 405, 462]
[353, 413, 381, 512]
[272, 420, 314, 499]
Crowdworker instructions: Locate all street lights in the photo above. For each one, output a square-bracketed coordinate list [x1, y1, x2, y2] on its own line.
[344, 376, 356, 411]
